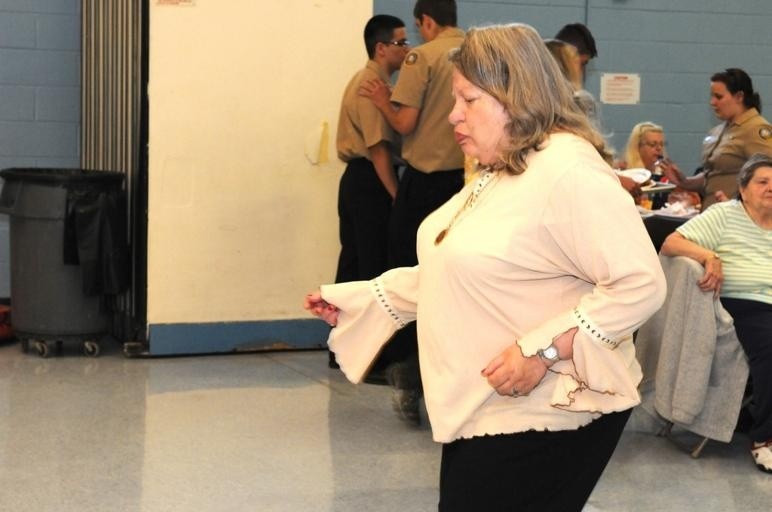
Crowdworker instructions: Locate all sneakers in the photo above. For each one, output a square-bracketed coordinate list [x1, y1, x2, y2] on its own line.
[750, 441, 771, 473]
[385, 362, 422, 430]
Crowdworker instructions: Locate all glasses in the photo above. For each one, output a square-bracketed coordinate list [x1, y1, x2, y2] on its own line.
[638, 138, 667, 150]
[381, 39, 410, 49]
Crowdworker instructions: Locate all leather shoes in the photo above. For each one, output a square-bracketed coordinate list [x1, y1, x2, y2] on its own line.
[327, 351, 389, 387]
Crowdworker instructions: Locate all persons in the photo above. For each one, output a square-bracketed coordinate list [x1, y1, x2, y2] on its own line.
[329, 1, 484, 429]
[543, 22, 772, 214]
[301, 22, 668, 512]
[661, 152, 772, 476]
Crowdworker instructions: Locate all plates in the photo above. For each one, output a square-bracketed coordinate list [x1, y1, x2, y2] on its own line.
[617, 167, 656, 188]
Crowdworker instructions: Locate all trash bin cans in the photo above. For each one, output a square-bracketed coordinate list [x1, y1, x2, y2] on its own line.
[1, 168, 127, 356]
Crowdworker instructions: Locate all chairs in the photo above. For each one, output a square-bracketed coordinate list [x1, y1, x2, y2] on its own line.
[658, 256, 755, 460]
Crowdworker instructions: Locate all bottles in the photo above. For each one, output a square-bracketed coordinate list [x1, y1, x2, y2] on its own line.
[653, 155, 665, 181]
